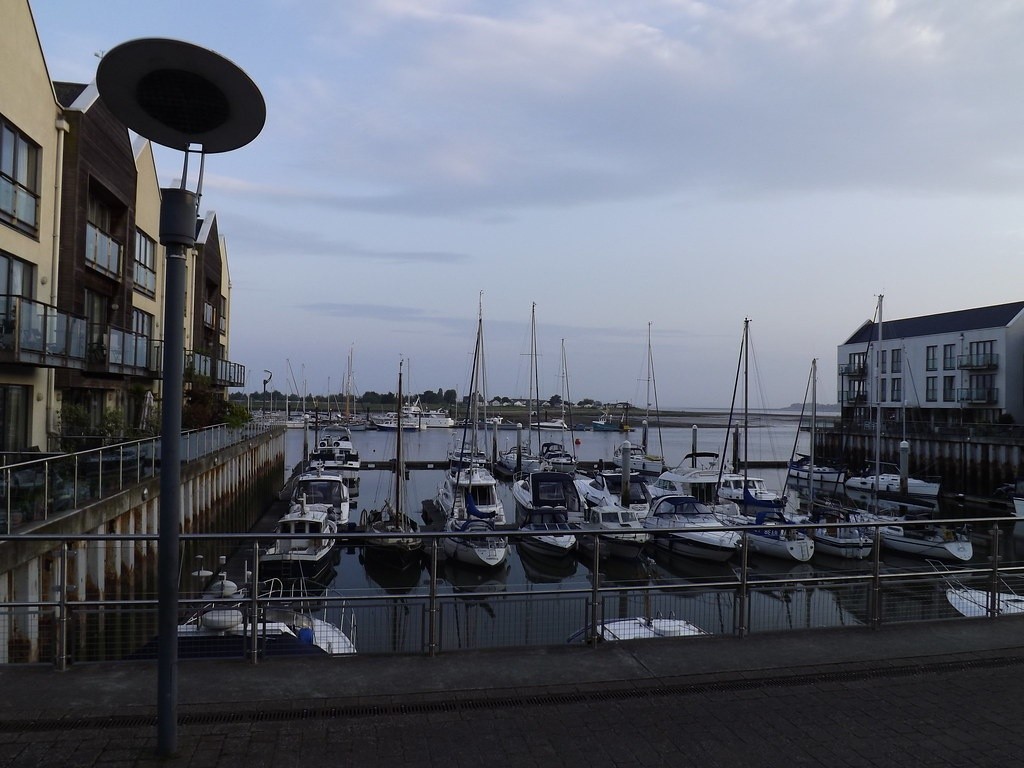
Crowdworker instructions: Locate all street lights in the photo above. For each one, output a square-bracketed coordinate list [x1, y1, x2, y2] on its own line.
[97, 36, 266, 768]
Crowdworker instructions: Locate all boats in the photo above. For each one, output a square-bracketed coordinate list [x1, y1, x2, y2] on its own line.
[569, 424, 591, 431]
[258, 486, 339, 579]
[946, 585, 1024, 616]
[530, 419, 569, 430]
[178, 553, 360, 656]
[288, 460, 351, 529]
[374, 420, 419, 430]
[478, 417, 523, 431]
[308, 420, 361, 478]
[592, 405, 635, 431]
[593, 616, 775, 633]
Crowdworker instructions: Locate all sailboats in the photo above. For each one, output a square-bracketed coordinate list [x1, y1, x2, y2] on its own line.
[248, 291, 1006, 580]
